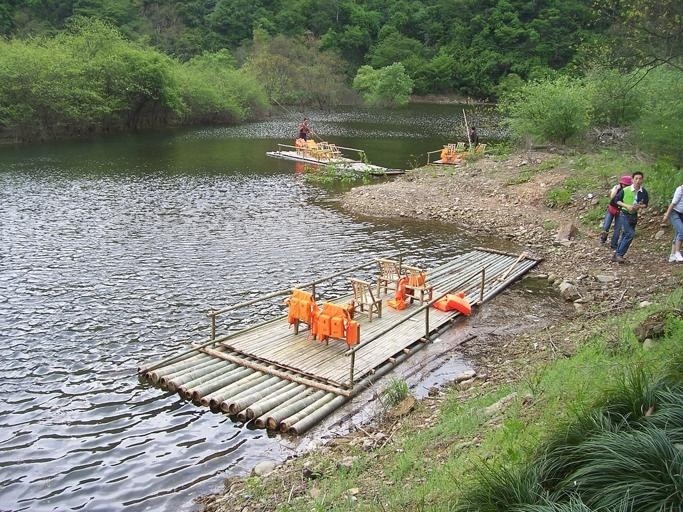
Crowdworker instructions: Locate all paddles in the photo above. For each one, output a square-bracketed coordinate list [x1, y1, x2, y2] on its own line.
[498, 251, 528, 282]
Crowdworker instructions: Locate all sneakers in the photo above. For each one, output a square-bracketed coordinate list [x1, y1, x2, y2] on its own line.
[611, 254, 626, 263]
[599, 230, 610, 243]
[668, 251, 683, 262]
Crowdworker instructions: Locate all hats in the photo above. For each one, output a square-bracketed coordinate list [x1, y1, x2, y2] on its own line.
[618, 175, 632, 186]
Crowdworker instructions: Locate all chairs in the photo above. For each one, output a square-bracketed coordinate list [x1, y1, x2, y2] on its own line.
[347, 257, 433, 322]
[295, 137, 341, 161]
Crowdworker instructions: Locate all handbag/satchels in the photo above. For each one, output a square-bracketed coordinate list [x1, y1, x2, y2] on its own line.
[606, 204, 620, 217]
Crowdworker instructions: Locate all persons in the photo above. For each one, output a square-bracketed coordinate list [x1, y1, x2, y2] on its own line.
[469, 127, 478, 146]
[661, 181, 683, 265]
[597, 175, 632, 248]
[297, 118, 309, 141]
[609, 172, 649, 264]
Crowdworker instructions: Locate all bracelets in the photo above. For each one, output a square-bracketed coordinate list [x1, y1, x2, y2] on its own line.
[663, 213, 669, 217]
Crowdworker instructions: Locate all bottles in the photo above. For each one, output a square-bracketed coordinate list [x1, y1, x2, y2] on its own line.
[633, 198, 643, 212]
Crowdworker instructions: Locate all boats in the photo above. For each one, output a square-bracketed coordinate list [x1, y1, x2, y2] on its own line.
[132, 247, 544, 438]
[265, 140, 407, 178]
[425, 142, 499, 167]
